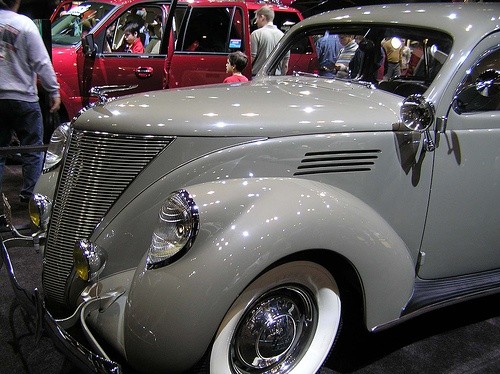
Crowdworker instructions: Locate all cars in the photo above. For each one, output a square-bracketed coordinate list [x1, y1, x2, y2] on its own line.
[3, 2, 500, 373]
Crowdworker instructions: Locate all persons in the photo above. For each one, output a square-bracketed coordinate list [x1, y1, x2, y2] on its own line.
[112, 14, 145, 53]
[316, 34, 410, 82]
[0, 0, 61, 200]
[223, 51, 248, 84]
[250, 6, 290, 76]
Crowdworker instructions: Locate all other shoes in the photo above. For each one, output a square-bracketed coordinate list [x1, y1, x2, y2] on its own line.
[19, 194, 32, 202]
[5, 154, 23, 165]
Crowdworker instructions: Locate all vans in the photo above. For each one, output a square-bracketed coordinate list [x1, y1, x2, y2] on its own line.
[42, 0, 319, 142]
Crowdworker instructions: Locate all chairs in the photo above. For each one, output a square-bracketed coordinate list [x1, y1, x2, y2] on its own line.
[145, 39, 161, 54]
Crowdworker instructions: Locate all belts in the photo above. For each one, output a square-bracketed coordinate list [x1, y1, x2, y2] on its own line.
[389, 61, 399, 64]
